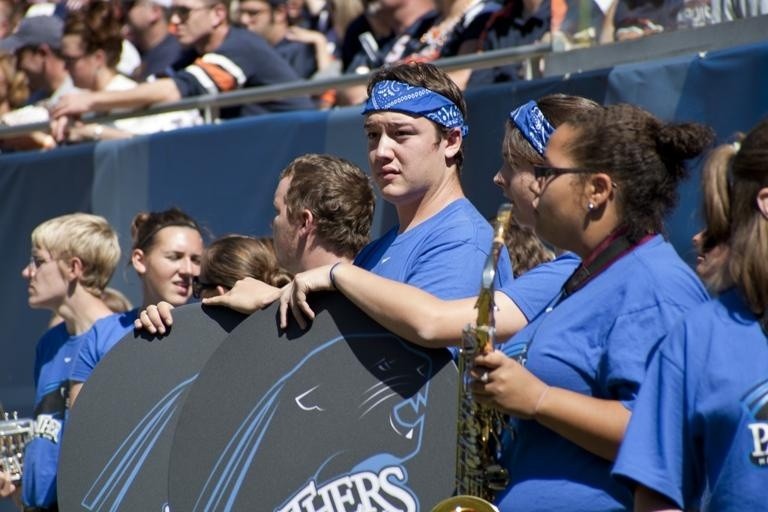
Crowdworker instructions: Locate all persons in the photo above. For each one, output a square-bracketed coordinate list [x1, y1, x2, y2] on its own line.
[68, 207, 203, 411]
[133, 62, 514, 335]
[610, 118, 768, 512]
[259, 93, 603, 349]
[472, 101, 711, 512]
[47, 287, 132, 330]
[191, 233, 280, 300]
[20, 212, 122, 512]
[1, 0, 768, 152]
[691, 217, 741, 294]
[272, 152, 376, 276]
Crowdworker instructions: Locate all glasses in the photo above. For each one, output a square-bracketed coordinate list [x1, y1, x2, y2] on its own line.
[61, 51, 91, 65]
[534, 165, 620, 190]
[170, 4, 214, 17]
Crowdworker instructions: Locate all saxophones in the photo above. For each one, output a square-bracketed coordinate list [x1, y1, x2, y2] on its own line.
[432, 202, 515, 512]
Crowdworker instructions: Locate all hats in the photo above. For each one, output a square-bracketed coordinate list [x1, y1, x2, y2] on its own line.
[2, 16, 64, 55]
